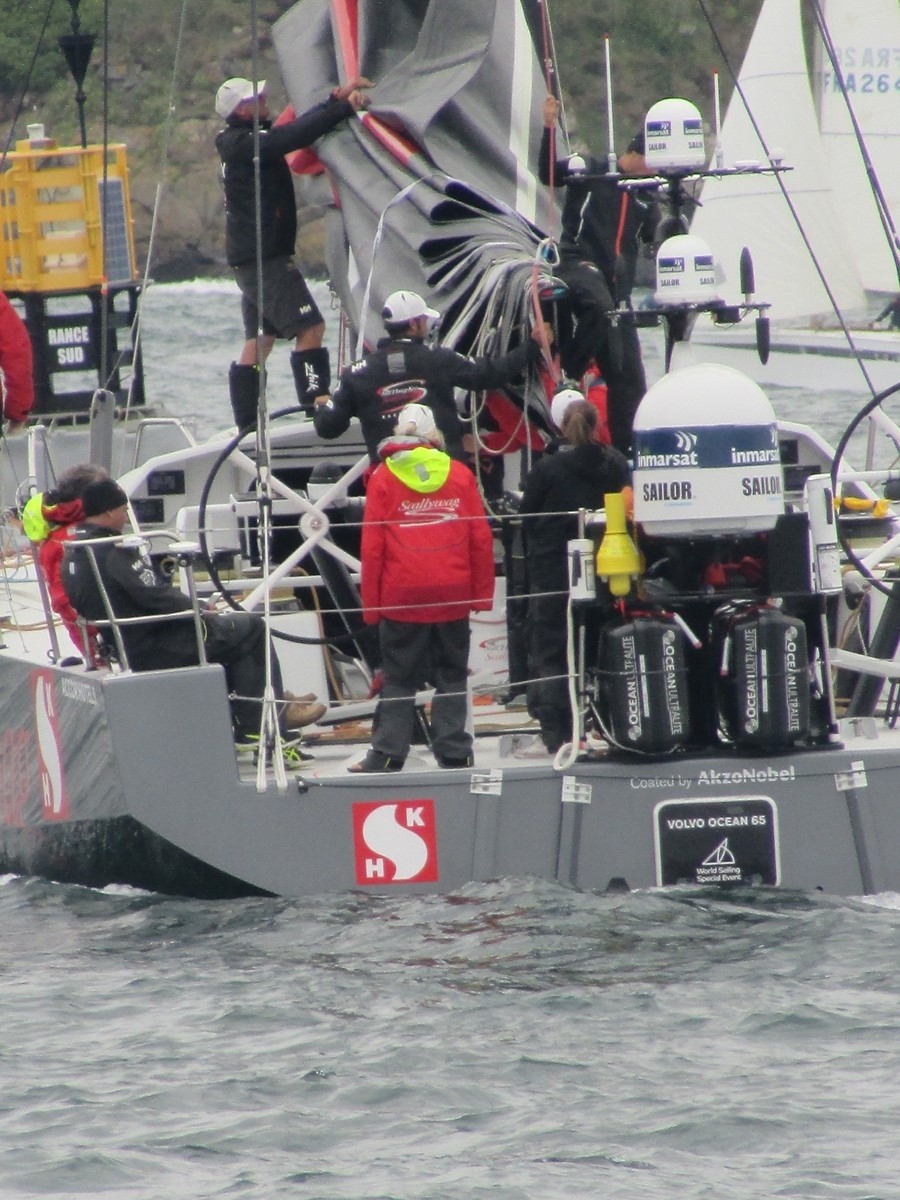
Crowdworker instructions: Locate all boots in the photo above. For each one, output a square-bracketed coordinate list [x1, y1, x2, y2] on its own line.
[228, 361, 269, 434]
[290, 348, 338, 421]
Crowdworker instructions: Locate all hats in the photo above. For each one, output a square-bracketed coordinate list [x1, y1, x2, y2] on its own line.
[551, 390, 585, 428]
[83, 478, 128, 515]
[398, 403, 435, 436]
[382, 290, 440, 322]
[215, 78, 266, 119]
[627, 129, 646, 154]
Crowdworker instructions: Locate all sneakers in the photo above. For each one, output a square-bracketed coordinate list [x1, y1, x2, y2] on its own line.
[234, 716, 302, 752]
[252, 747, 317, 769]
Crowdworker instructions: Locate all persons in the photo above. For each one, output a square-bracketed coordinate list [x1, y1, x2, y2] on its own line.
[38, 465, 327, 769]
[534, 92, 661, 469]
[312, 288, 631, 773]
[0, 290, 34, 438]
[214, 76, 374, 439]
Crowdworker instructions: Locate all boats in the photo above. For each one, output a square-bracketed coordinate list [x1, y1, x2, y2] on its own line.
[0, 0, 899, 909]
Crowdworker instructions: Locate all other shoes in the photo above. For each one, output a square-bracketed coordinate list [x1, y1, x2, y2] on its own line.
[283, 691, 316, 704]
[347, 755, 400, 773]
[437, 755, 474, 768]
[284, 704, 326, 728]
